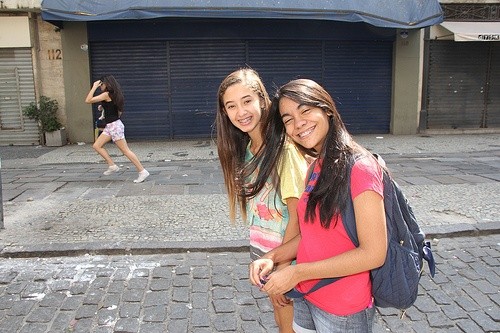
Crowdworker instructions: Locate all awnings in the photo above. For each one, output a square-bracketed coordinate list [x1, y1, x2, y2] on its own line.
[39, 0, 500, 42]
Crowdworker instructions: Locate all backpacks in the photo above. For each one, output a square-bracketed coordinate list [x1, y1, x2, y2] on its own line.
[305, 152, 436, 309]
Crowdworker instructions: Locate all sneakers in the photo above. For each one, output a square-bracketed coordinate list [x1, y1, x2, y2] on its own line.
[134, 170, 150, 183]
[104, 165, 120, 175]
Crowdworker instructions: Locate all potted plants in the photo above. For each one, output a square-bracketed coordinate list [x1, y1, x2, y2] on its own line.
[21, 95, 67, 147]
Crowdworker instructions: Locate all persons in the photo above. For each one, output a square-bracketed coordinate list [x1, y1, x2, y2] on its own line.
[252, 79, 388, 333]
[85, 76, 151, 183]
[216, 67, 307, 333]
